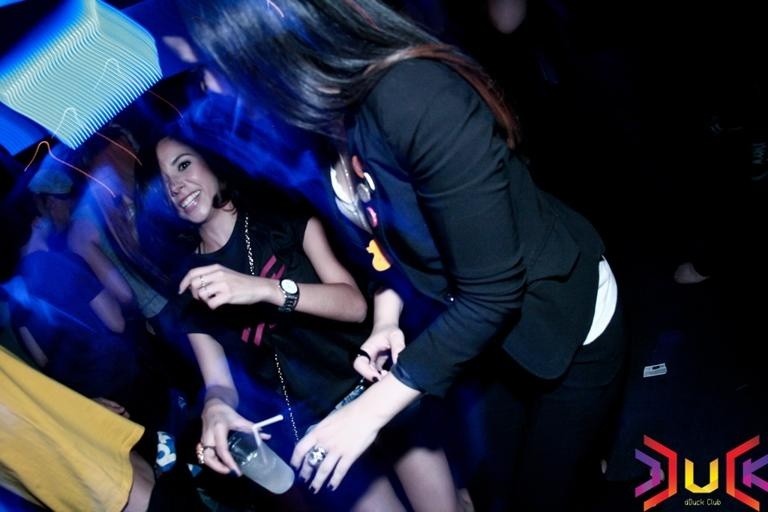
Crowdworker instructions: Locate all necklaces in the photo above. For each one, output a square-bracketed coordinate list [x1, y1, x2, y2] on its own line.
[338, 151, 367, 231]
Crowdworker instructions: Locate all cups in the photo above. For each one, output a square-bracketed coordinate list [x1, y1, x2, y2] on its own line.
[225, 430, 295, 495]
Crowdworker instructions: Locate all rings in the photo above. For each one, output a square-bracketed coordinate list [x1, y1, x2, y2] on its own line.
[197, 443, 215, 463]
[308, 447, 326, 467]
[357, 349, 371, 360]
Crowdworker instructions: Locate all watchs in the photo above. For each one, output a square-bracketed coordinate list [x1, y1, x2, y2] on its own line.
[278, 278, 299, 312]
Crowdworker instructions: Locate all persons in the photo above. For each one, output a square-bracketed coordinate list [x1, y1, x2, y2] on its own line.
[137, 126, 473, 512]
[155, 0, 622, 512]
[0, 127, 212, 512]
